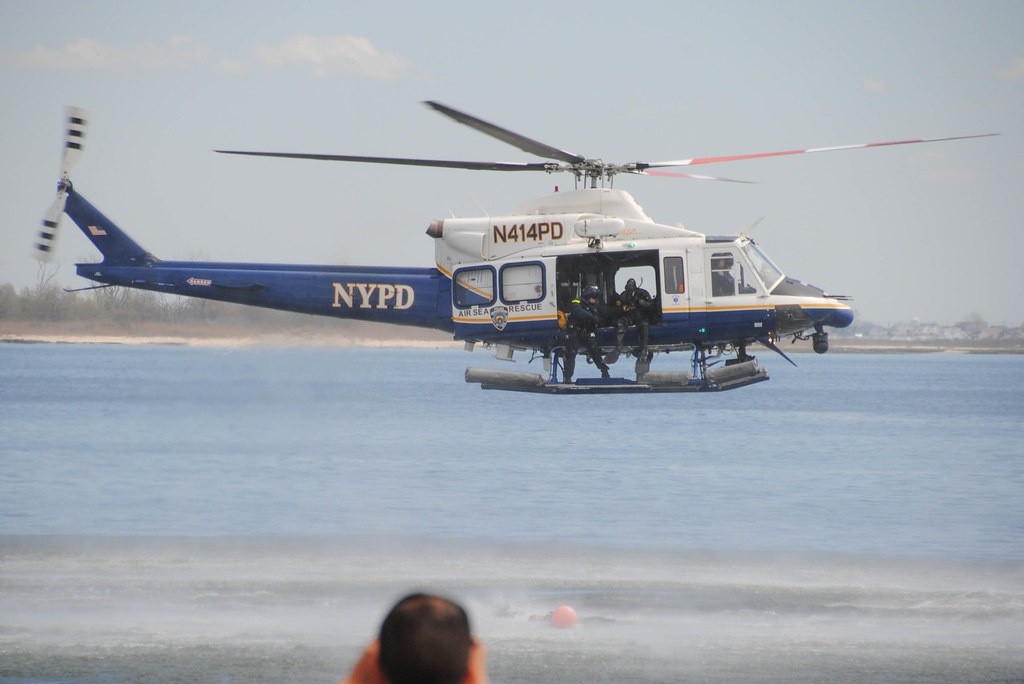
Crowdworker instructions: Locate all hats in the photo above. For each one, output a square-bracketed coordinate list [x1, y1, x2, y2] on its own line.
[625, 278, 636, 292]
[581, 287, 598, 301]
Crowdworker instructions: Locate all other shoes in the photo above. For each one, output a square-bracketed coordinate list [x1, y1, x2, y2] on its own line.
[563, 377, 571, 384]
[602, 372, 610, 379]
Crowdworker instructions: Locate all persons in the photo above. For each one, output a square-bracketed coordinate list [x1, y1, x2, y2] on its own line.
[563, 276, 654, 384]
[337, 592, 488, 684]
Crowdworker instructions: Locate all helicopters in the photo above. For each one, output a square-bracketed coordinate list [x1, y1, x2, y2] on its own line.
[28, 98, 1001, 392]
